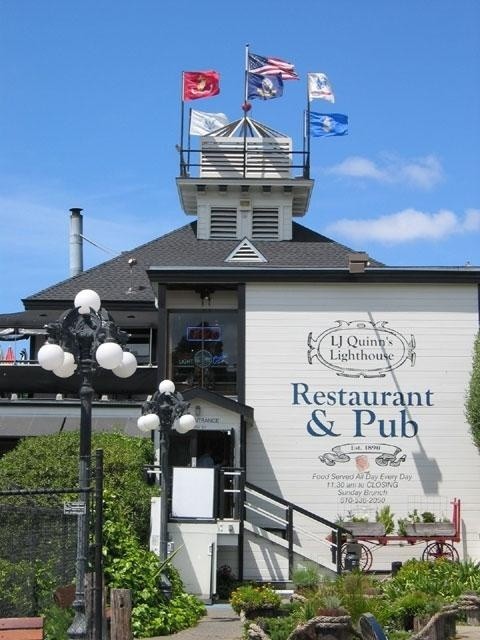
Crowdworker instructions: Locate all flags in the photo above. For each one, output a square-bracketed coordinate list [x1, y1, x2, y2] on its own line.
[248, 51, 300, 80]
[306, 109, 348, 138]
[307, 71, 336, 104]
[183, 70, 220, 101]
[247, 71, 284, 100]
[189, 108, 229, 137]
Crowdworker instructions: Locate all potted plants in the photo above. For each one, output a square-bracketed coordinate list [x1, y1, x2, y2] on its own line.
[334, 501, 395, 536]
[398, 509, 455, 536]
[229, 556, 480, 640]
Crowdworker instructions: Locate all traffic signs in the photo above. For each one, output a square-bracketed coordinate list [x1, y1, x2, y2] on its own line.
[63, 501, 86, 515]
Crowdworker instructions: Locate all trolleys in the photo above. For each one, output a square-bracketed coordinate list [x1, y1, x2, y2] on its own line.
[325, 495, 470, 576]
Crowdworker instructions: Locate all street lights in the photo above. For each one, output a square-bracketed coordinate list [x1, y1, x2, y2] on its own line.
[33, 283, 146, 638]
[131, 373, 197, 606]
